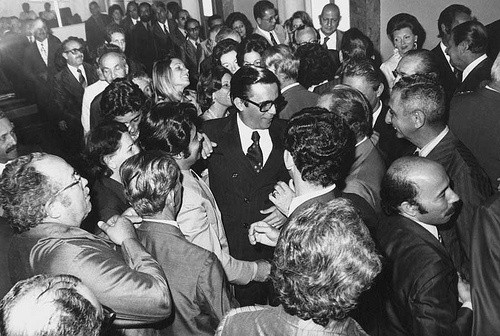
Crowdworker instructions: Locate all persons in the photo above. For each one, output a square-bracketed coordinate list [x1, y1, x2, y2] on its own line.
[0, 1, 500, 336]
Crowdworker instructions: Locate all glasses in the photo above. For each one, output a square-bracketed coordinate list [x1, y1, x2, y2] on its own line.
[179, 16, 190, 20]
[239, 96, 284, 112]
[52, 170, 82, 196]
[262, 15, 279, 22]
[392, 70, 410, 78]
[66, 47, 86, 54]
[243, 59, 261, 68]
[92, 64, 101, 70]
[189, 26, 200, 32]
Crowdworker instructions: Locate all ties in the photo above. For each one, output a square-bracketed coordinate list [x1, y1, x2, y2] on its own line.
[413, 151, 420, 157]
[40, 44, 48, 66]
[270, 32, 278, 46]
[185, 31, 187, 35]
[136, 19, 140, 24]
[322, 37, 330, 50]
[77, 69, 86, 88]
[163, 24, 168, 34]
[195, 40, 198, 46]
[245, 131, 263, 174]
[146, 22, 150, 31]
